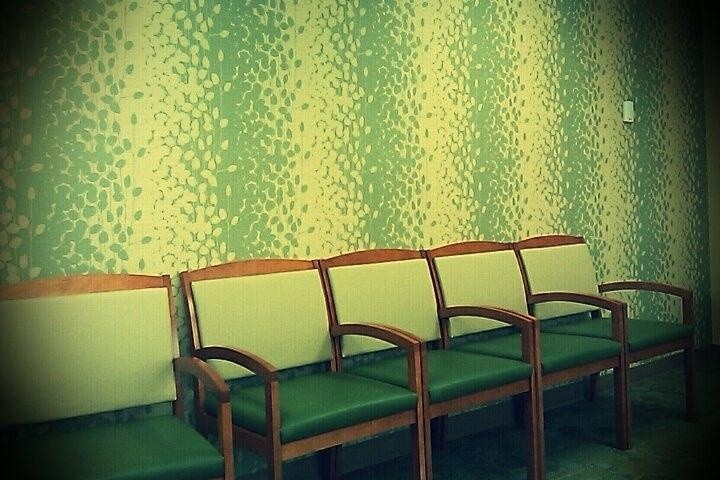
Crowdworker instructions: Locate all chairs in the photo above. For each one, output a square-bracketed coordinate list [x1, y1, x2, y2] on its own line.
[178, 257, 427, 480]
[320, 245, 541, 480]
[515, 234, 697, 449]
[1, 268, 239, 480]
[427, 239, 628, 478]
